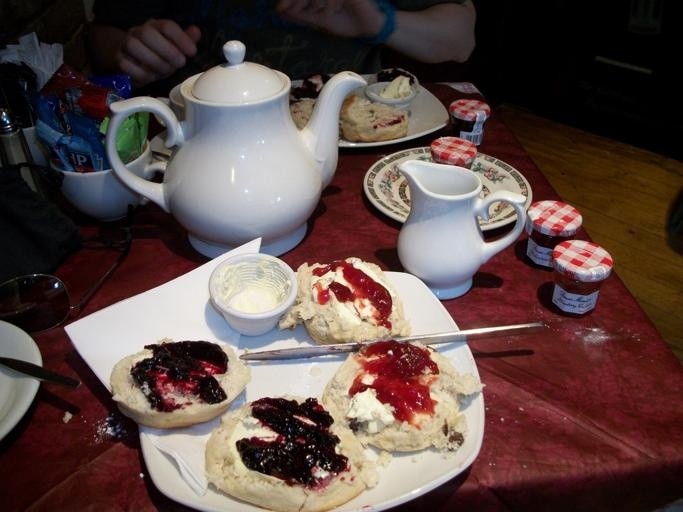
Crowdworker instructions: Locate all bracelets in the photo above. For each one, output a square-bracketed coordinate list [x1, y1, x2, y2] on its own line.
[363, 2, 396, 46]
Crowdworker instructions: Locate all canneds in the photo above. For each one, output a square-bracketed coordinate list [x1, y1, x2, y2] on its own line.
[430, 137, 478, 169]
[447, 99, 490, 147]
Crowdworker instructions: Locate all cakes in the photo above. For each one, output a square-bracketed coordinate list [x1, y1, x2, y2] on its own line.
[206, 394, 377, 511]
[322, 338, 486, 452]
[280, 257, 411, 345]
[289, 68, 419, 142]
[109, 338, 251, 428]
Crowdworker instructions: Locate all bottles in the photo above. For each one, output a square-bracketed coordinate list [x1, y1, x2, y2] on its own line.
[450, 97, 494, 145]
[433, 134, 476, 168]
[1, 107, 34, 165]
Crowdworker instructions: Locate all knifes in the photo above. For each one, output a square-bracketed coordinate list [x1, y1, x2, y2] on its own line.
[240, 320, 547, 361]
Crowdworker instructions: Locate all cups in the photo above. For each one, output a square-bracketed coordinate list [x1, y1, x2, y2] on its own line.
[207, 251, 300, 339]
[365, 82, 416, 114]
[47, 137, 166, 221]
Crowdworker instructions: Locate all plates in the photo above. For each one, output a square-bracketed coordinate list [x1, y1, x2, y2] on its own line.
[0, 321, 50, 439]
[287, 70, 450, 148]
[361, 142, 534, 229]
[138, 273, 487, 510]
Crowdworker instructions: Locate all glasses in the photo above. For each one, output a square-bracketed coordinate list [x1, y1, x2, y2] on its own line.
[0, 230, 134, 334]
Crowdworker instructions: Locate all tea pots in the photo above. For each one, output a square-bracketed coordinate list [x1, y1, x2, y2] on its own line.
[397, 158, 529, 299]
[101, 39, 369, 262]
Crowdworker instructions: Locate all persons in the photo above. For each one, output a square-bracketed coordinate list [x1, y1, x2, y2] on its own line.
[84, 0, 477, 89]
